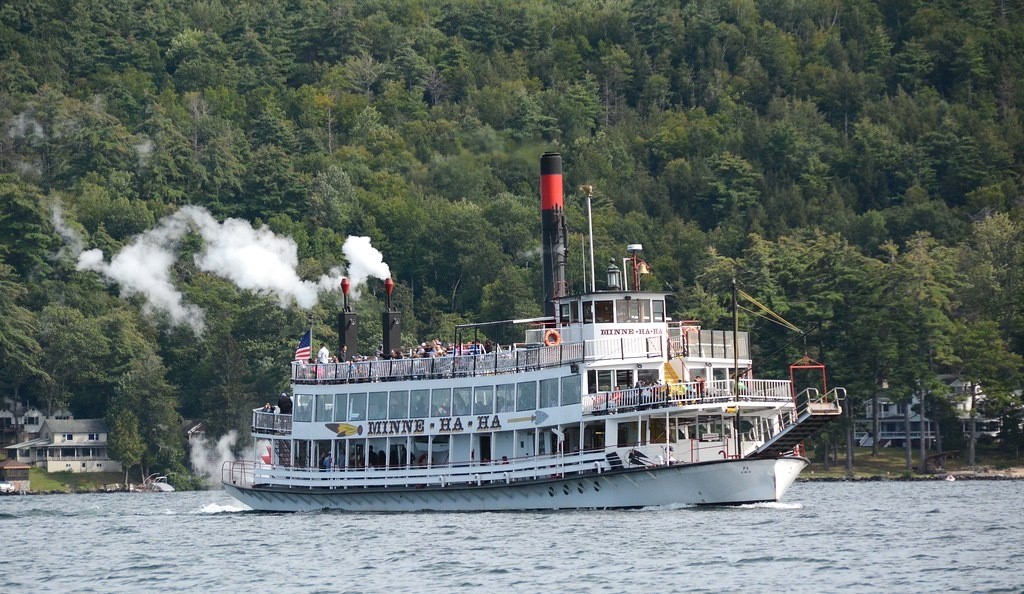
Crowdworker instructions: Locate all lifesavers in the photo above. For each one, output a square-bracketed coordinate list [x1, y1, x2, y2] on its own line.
[683, 327, 699, 344]
[545, 330, 561, 346]
[418, 453, 435, 469]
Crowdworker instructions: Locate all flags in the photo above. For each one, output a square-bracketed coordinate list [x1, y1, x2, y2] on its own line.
[295, 330, 310, 360]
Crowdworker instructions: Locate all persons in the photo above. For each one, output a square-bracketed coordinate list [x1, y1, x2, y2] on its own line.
[400, 447, 416, 486]
[363, 445, 386, 488]
[608, 376, 705, 414]
[318, 343, 329, 364]
[793, 445, 799, 456]
[319, 451, 335, 489]
[731, 370, 751, 400]
[338, 345, 347, 363]
[296, 338, 494, 385]
[260, 392, 293, 433]
[483, 456, 511, 484]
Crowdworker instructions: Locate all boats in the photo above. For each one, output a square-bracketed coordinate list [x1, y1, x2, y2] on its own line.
[136, 477, 175, 492]
[221, 151, 847, 512]
